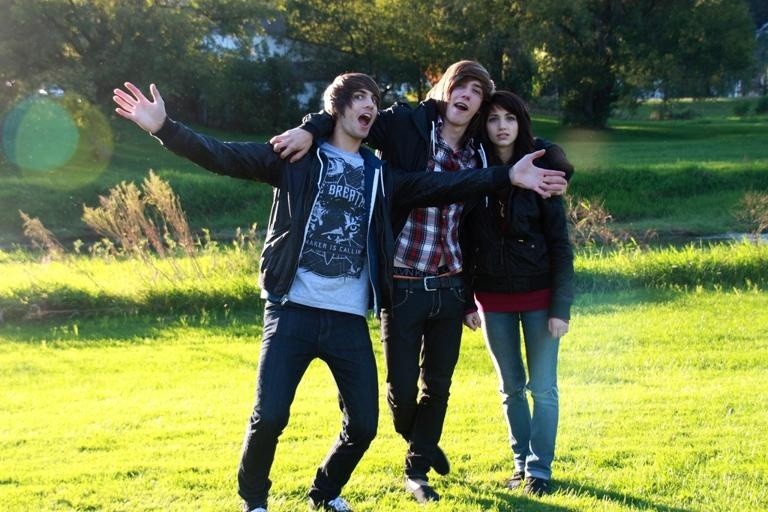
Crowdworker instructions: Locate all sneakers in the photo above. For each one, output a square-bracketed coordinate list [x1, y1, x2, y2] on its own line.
[524, 475, 550, 498]
[308, 496, 351, 512]
[508, 473, 523, 490]
[242, 503, 268, 512]
[431, 445, 449, 475]
[405, 479, 439, 504]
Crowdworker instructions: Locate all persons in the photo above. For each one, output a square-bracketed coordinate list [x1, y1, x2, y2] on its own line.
[270, 60, 575, 503]
[456, 92, 576, 493]
[113, 73, 566, 511]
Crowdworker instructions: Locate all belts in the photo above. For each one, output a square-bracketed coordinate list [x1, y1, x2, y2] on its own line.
[392, 274, 463, 290]
